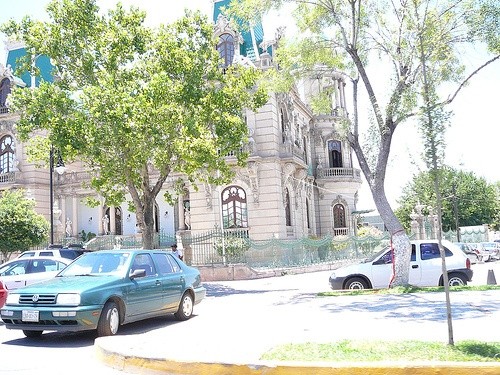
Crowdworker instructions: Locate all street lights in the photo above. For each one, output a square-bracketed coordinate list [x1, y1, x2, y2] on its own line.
[48, 143, 65, 248]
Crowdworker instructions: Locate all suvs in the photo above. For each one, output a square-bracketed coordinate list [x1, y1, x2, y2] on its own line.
[328, 239, 473, 291]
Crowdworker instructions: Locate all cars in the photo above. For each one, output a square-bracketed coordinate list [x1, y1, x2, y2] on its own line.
[452, 242, 500, 264]
[0, 248, 92, 275]
[0, 256, 98, 291]
[0, 248, 206, 339]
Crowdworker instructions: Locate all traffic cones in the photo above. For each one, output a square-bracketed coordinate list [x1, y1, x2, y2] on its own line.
[486, 269, 497, 285]
